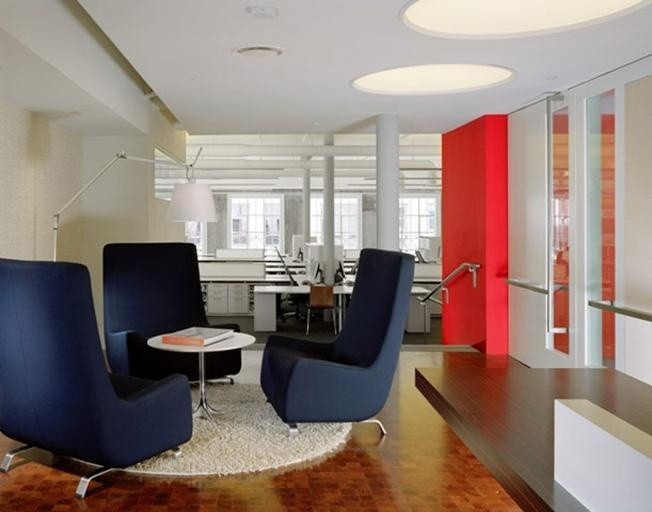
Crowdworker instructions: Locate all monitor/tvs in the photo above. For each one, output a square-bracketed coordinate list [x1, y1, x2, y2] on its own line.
[313, 262, 322, 283]
[335, 261, 346, 283]
[297, 247, 303, 262]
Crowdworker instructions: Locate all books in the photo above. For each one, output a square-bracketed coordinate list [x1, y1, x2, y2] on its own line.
[162, 327, 234, 347]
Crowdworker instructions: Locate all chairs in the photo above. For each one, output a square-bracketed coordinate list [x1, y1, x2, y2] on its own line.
[0, 257, 193, 499]
[101, 243, 242, 384]
[259, 248, 415, 436]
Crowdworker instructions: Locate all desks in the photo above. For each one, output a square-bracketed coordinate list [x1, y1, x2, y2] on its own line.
[197, 254, 357, 336]
[332, 282, 433, 338]
[147, 330, 256, 422]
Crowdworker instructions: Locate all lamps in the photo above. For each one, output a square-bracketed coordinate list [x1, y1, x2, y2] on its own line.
[49, 151, 219, 265]
